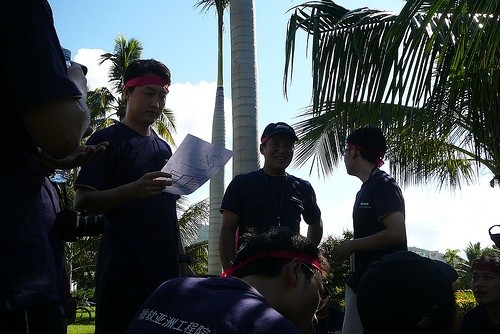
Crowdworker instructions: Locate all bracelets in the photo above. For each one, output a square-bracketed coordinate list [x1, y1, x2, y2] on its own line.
[179, 254, 192, 266]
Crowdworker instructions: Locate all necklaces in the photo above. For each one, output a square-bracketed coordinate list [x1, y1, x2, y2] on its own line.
[126, 136, 163, 162]
[261, 167, 288, 221]
[353, 166, 377, 216]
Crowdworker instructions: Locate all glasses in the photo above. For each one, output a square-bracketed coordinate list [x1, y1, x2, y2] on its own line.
[301, 260, 334, 313]
[342, 147, 351, 155]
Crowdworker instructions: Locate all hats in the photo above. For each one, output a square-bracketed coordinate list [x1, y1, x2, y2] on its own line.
[262, 121, 300, 141]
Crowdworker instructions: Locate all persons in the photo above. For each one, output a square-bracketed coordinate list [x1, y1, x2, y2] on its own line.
[219, 122, 323, 272]
[72, 58, 197, 334]
[315, 248, 500, 334]
[1, 0, 109, 334]
[125, 226, 330, 334]
[330, 126, 408, 334]
